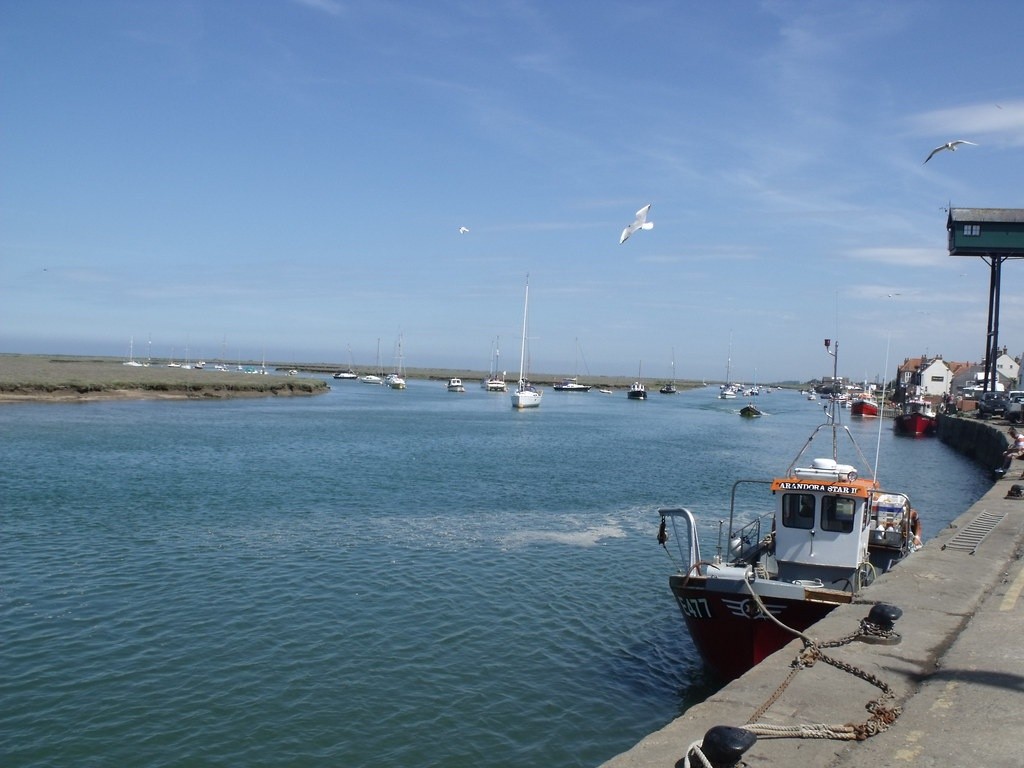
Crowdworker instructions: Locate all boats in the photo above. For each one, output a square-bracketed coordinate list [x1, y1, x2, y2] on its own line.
[800, 382, 856, 410]
[659, 336, 921, 680]
[894, 366, 938, 437]
[446, 376, 465, 392]
[599, 389, 612, 394]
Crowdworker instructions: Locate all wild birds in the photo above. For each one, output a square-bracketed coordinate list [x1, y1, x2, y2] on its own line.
[458, 226, 470, 235]
[923, 140, 979, 164]
[618, 204, 654, 245]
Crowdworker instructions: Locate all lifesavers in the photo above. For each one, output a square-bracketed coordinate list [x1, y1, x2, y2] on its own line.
[903, 508, 922, 547]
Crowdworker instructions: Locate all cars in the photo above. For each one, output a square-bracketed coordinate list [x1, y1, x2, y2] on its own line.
[978, 392, 1009, 418]
[1006, 390, 1024, 420]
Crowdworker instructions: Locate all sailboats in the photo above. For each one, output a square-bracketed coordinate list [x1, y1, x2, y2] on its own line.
[361, 331, 414, 391]
[480, 341, 493, 388]
[627, 360, 647, 400]
[739, 368, 763, 416]
[486, 336, 507, 392]
[719, 356, 785, 400]
[333, 343, 356, 379]
[660, 368, 676, 394]
[120, 324, 304, 380]
[553, 337, 592, 392]
[511, 274, 543, 409]
[851, 370, 878, 416]
